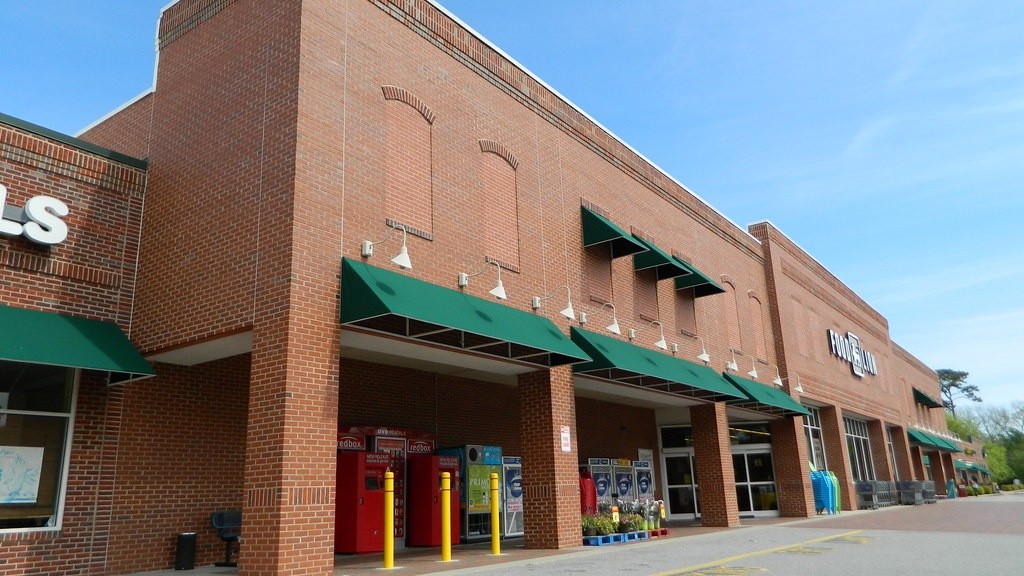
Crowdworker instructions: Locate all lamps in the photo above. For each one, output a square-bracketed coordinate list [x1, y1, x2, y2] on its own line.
[776, 372, 803, 393]
[580, 303, 621, 335]
[706, 347, 738, 371]
[361, 225, 413, 269]
[757, 364, 783, 386]
[727, 355, 757, 378]
[628, 321, 667, 351]
[672, 335, 710, 363]
[532, 285, 575, 320]
[459, 261, 506, 300]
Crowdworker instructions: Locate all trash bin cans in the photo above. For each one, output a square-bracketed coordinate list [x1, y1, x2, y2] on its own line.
[945, 479, 957, 498]
[175, 532, 196, 570]
[957, 479, 966, 497]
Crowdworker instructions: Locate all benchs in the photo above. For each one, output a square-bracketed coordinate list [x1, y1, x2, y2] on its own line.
[211, 511, 243, 566]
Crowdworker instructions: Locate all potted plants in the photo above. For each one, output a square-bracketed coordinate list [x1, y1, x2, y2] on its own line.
[582, 499, 661, 536]
